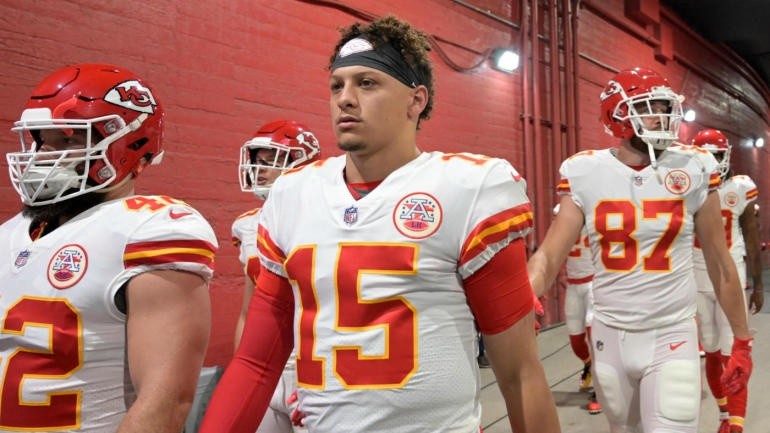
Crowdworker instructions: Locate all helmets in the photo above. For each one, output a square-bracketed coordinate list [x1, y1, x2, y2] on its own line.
[6, 62, 165, 206]
[238, 120, 319, 200]
[599, 66, 688, 150]
[692, 129, 732, 177]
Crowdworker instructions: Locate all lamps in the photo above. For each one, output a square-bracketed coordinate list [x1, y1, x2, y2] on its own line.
[681, 108, 696, 122]
[489, 47, 521, 74]
[751, 132, 766, 149]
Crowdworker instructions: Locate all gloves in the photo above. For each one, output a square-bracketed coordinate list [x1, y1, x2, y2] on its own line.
[532, 293, 548, 335]
[720, 337, 754, 396]
[287, 393, 306, 427]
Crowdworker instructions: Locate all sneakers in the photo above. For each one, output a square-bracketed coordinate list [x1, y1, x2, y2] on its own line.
[588, 394, 602, 413]
[579, 362, 591, 388]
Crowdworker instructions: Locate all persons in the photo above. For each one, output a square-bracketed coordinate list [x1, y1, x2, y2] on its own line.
[527, 68, 754, 433]
[231, 119, 320, 433]
[198, 15, 561, 433]
[0, 64, 217, 433]
[552, 202, 604, 413]
[693, 129, 765, 433]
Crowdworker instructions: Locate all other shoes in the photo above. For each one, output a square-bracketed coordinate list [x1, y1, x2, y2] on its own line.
[719, 422, 730, 433]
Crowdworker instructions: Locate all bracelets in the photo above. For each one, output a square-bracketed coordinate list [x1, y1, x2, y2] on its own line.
[753, 290, 763, 293]
